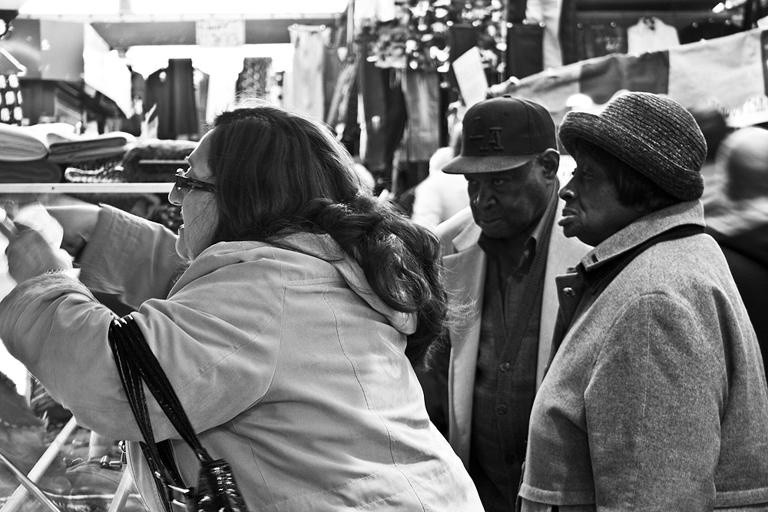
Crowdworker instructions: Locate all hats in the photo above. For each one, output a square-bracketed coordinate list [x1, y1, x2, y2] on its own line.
[443, 95, 555, 174]
[559, 92, 708, 201]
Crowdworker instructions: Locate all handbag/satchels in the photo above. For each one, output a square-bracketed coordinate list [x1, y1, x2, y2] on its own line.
[150, 456, 249, 512]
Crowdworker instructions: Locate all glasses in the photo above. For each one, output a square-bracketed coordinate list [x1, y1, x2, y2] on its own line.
[174, 168, 217, 196]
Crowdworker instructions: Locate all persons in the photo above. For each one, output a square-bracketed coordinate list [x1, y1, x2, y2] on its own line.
[511, 91, 767, 510]
[406, 91, 768, 381]
[404, 94, 594, 512]
[0, 106, 489, 511]
